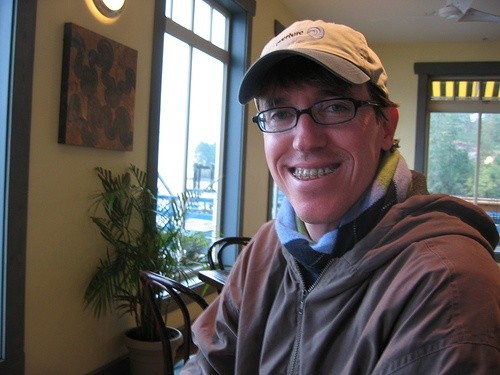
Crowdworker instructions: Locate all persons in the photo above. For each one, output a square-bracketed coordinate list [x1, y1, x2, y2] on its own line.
[179, 19, 500, 375]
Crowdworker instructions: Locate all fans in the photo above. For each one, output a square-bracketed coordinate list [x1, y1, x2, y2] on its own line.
[372, 0, 500, 24]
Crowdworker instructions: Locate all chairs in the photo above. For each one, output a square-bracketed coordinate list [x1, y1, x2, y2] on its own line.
[140, 268, 210, 375]
[208, 236, 252, 293]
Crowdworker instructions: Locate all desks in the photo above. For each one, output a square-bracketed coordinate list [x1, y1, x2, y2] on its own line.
[198, 270, 230, 291]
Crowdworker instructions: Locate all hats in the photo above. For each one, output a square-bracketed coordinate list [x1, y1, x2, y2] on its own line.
[238, 18, 390, 105]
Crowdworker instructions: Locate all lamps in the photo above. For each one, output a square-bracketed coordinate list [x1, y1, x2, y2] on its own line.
[85, 0, 126, 25]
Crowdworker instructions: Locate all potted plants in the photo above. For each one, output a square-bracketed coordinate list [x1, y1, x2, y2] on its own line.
[80, 163, 211, 375]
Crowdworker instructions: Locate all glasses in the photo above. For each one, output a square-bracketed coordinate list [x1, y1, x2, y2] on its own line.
[252, 97, 382, 133]
